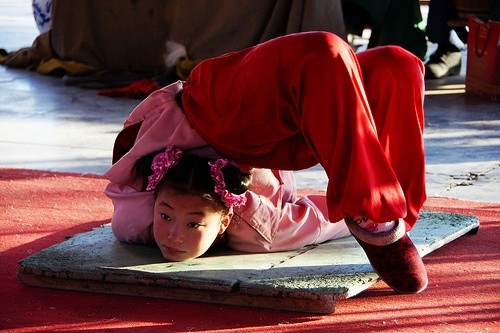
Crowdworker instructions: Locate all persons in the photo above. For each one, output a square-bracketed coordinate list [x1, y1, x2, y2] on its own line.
[102, 31, 429, 295]
[425, 0, 461, 78]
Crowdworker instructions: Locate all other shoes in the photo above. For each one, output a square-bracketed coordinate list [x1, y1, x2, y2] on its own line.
[344, 217, 428, 292]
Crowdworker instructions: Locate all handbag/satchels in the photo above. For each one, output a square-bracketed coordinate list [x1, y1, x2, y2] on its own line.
[466, 15, 500, 103]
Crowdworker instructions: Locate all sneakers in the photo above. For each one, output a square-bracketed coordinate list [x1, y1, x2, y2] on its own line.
[424, 44, 462, 80]
